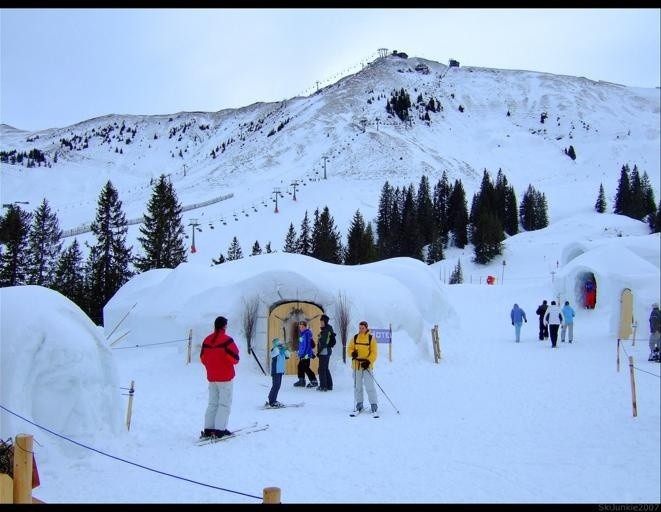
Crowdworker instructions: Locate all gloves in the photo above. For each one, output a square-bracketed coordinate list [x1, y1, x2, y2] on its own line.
[352, 352, 358, 358]
[361, 360, 370, 369]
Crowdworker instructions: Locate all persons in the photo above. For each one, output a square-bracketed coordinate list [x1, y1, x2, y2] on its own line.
[584, 277, 597, 309]
[560, 301, 576, 343]
[537, 300, 550, 341]
[294, 320, 319, 387]
[200, 316, 240, 437]
[544, 301, 565, 347]
[268, 337, 291, 407]
[648, 303, 661, 360]
[316, 314, 336, 391]
[349, 321, 379, 413]
[511, 303, 528, 343]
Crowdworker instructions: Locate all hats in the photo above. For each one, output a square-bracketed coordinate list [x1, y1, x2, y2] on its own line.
[361, 321, 368, 328]
[321, 315, 329, 322]
[299, 321, 306, 326]
[272, 338, 283, 348]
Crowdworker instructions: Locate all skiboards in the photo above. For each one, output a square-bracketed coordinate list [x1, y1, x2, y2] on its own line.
[257, 401, 304, 411]
[196, 420, 266, 448]
[350, 403, 381, 418]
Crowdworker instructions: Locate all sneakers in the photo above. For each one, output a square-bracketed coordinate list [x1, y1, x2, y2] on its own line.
[204, 429, 231, 438]
[316, 386, 333, 390]
[269, 401, 284, 407]
[371, 404, 377, 410]
[356, 402, 363, 409]
[307, 381, 318, 387]
[294, 381, 306, 387]
